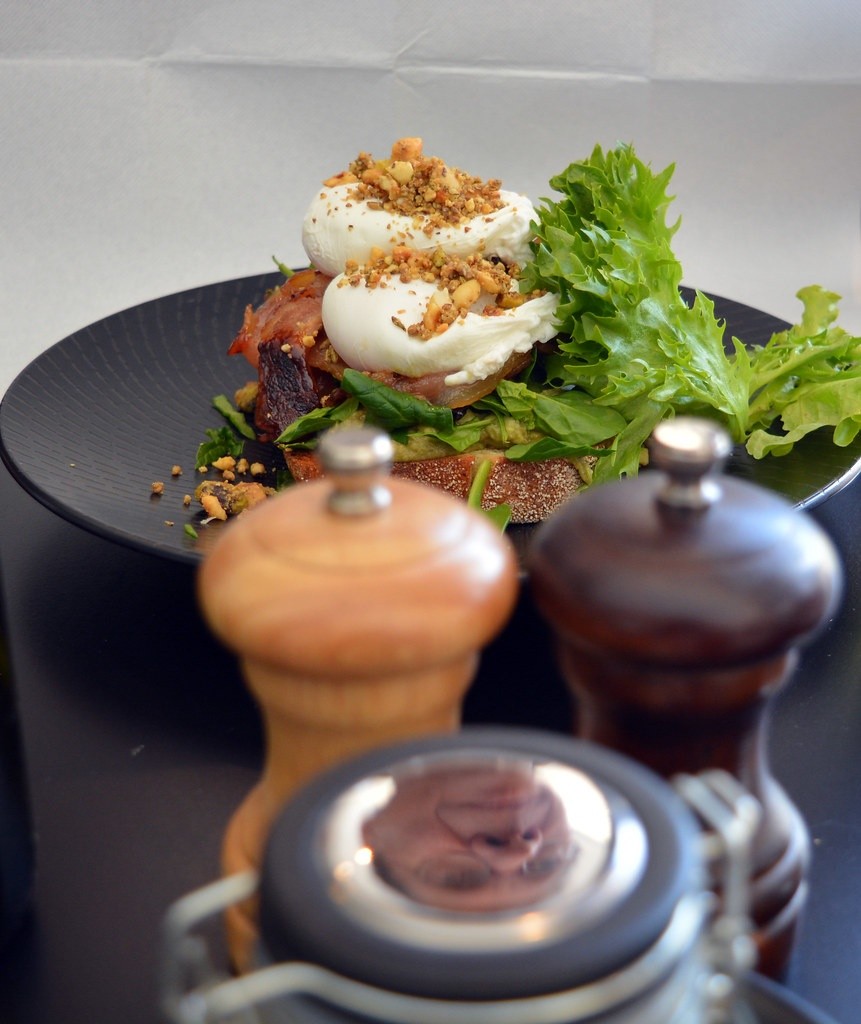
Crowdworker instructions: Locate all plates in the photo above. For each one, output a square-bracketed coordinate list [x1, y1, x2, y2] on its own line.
[0, 265, 861, 570]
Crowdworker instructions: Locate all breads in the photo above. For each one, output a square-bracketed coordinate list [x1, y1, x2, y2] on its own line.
[283, 449, 601, 522]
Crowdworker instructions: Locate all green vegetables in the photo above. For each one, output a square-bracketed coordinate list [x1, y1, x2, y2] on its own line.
[197, 142, 861, 537]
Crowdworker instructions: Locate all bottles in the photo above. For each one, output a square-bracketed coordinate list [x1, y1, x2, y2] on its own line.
[197, 428, 521, 976]
[521, 415, 845, 987]
